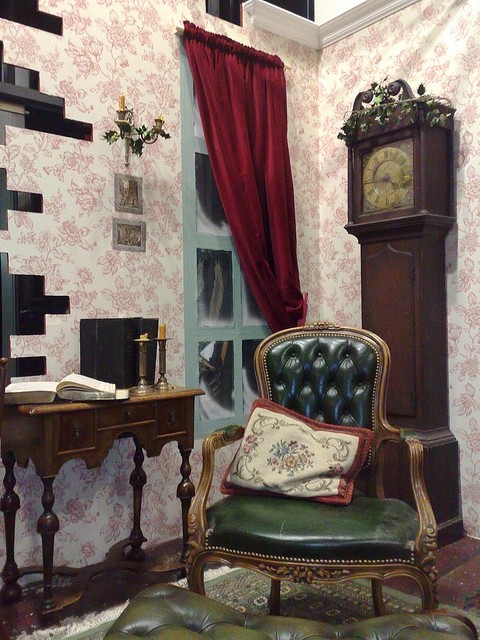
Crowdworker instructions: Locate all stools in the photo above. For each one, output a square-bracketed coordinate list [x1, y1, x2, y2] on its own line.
[102, 584, 475, 640]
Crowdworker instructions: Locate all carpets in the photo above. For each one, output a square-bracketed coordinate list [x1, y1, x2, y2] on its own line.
[16, 566, 479, 640]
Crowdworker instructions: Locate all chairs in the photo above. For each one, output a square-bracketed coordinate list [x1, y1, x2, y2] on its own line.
[185, 323, 439, 610]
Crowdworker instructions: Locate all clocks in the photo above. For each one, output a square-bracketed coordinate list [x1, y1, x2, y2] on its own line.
[353, 135, 416, 218]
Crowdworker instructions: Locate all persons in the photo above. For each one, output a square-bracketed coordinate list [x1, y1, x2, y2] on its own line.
[2, 372, 128, 409]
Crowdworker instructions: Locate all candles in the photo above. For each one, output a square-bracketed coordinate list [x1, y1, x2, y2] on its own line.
[140, 333, 149, 338]
[159, 322, 166, 339]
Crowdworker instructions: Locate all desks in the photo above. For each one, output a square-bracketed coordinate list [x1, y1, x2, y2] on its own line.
[1, 386, 205, 608]
